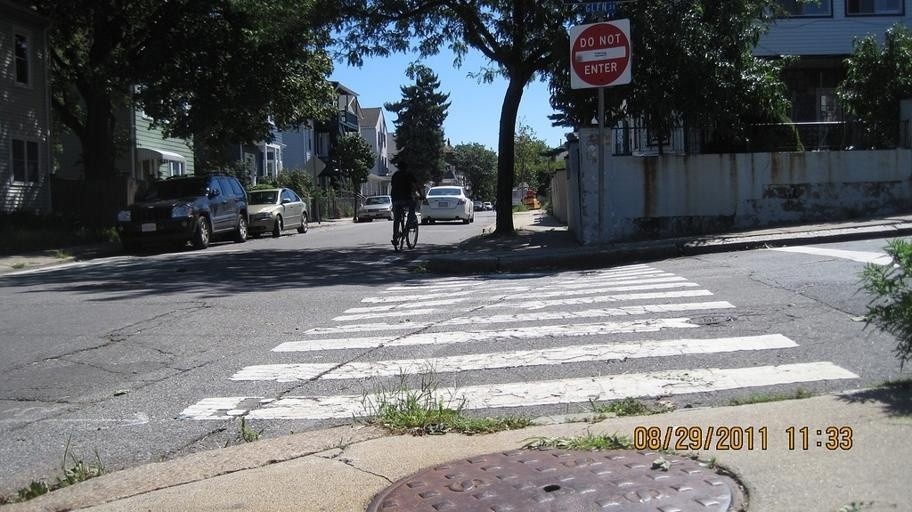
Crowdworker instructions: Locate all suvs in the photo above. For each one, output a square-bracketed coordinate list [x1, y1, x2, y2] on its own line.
[114, 169, 251, 256]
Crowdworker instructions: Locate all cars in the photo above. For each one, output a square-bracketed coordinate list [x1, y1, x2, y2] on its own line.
[473, 200, 495, 211]
[243, 186, 311, 238]
[419, 185, 474, 225]
[356, 193, 395, 222]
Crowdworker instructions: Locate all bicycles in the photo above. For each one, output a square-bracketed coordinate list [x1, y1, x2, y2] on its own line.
[390, 195, 426, 252]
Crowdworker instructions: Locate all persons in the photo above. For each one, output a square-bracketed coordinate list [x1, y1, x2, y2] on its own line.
[390, 161, 429, 239]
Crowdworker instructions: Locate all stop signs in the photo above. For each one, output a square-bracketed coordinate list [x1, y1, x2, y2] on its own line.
[570, 20, 630, 86]
